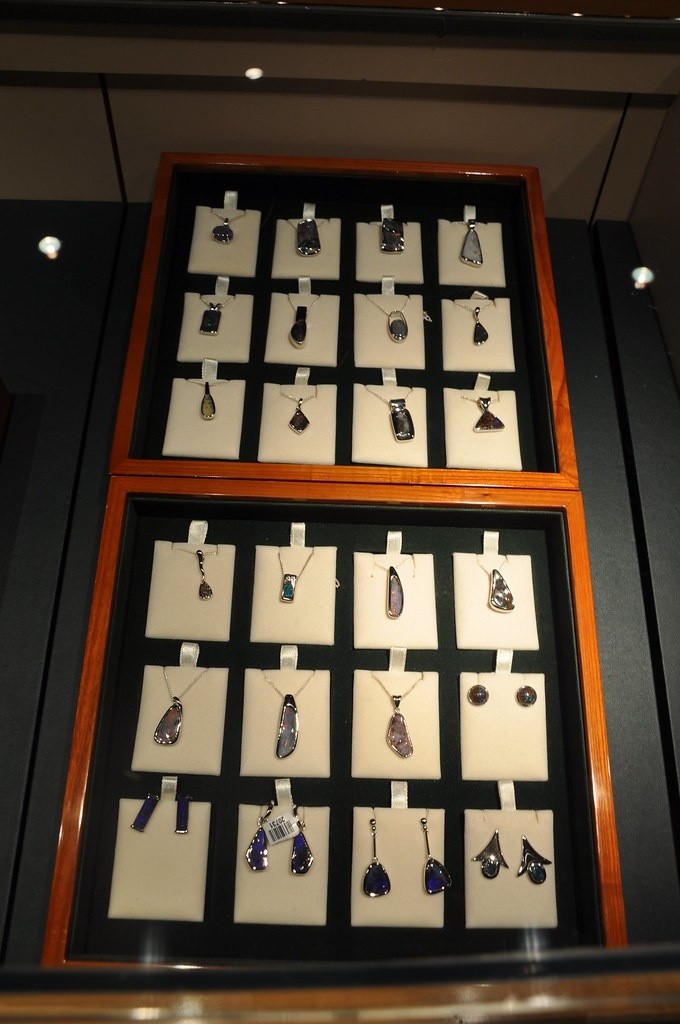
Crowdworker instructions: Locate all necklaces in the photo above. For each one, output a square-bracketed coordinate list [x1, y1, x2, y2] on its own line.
[210, 204, 489, 267]
[152, 663, 425, 760]
[196, 291, 496, 345]
[184, 377, 508, 442]
[169, 541, 515, 614]
